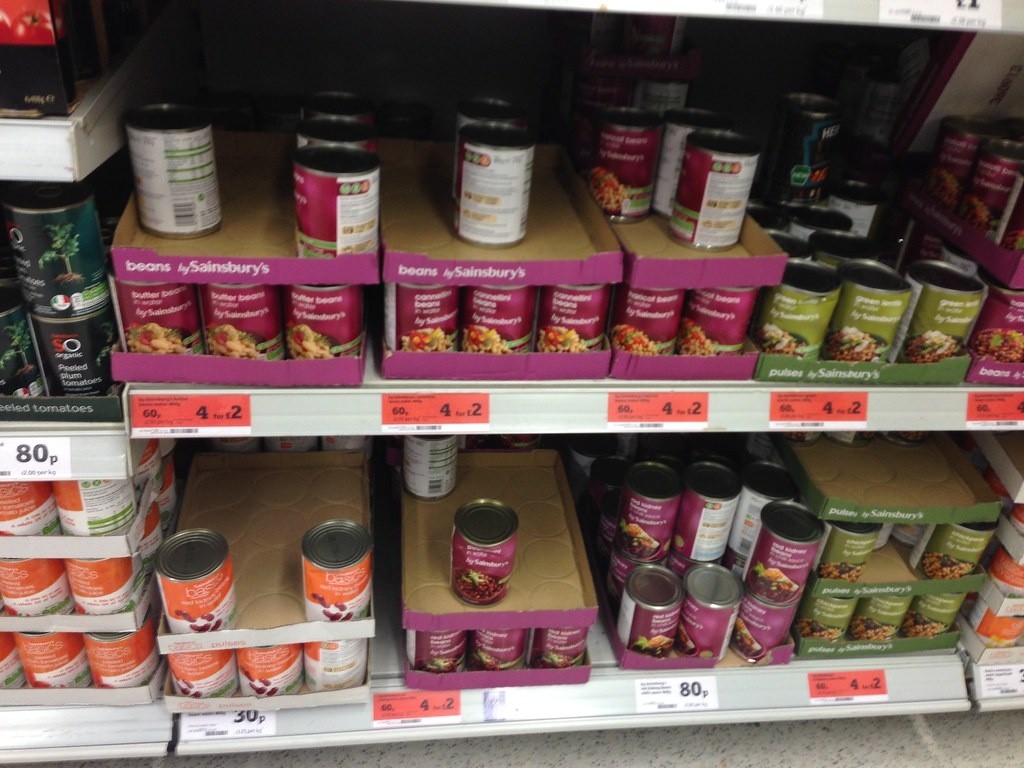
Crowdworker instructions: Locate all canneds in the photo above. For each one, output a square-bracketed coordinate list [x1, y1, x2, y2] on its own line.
[0, 91, 1024, 699]
